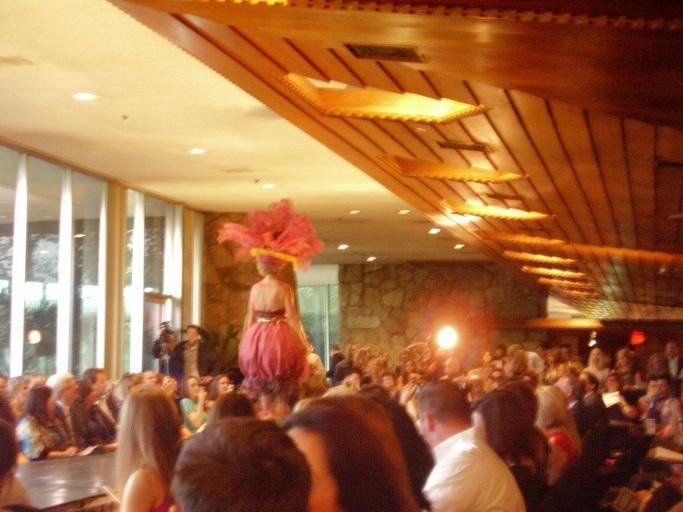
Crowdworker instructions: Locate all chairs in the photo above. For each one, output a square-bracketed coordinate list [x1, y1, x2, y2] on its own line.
[553, 414, 655, 512]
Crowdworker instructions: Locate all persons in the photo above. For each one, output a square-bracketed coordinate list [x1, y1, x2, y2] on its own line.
[235, 253, 316, 422]
[121, 324, 419, 512]
[324, 331, 681, 512]
[1, 368, 121, 512]
